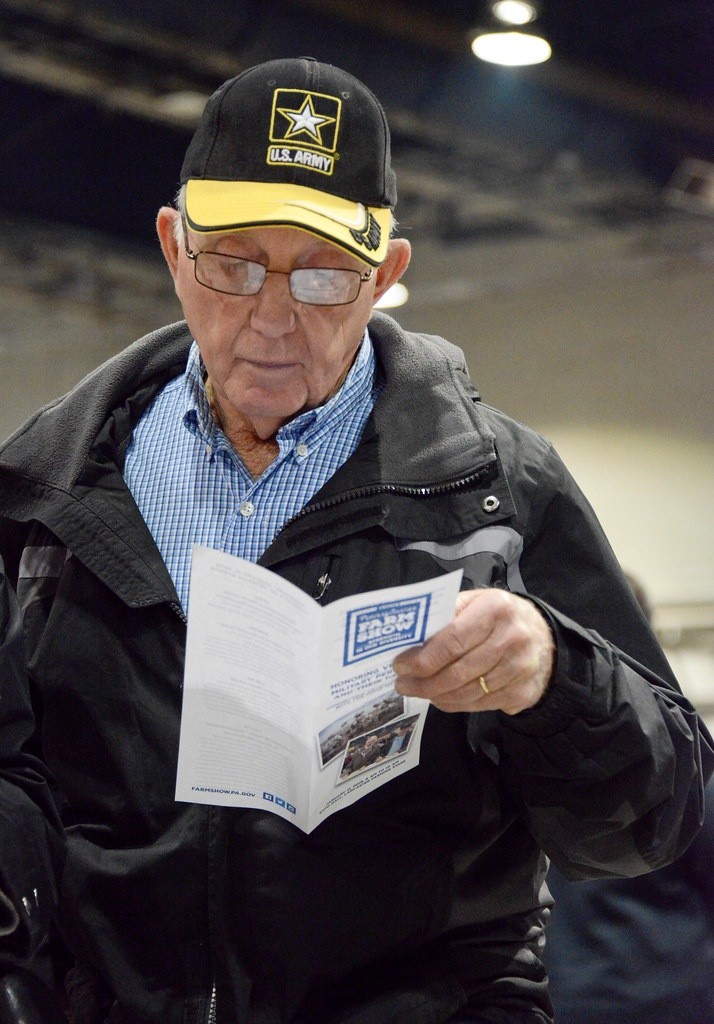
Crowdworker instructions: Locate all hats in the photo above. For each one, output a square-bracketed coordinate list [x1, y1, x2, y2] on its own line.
[179, 54, 397, 266]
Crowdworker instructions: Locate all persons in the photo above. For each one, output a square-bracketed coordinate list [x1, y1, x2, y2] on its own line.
[1, 56, 713, 1024]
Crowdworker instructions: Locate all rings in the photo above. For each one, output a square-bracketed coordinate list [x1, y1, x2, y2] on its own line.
[479, 677, 490, 695]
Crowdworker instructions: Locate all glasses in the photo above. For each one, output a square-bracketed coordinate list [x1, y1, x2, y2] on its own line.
[178, 215, 375, 308]
